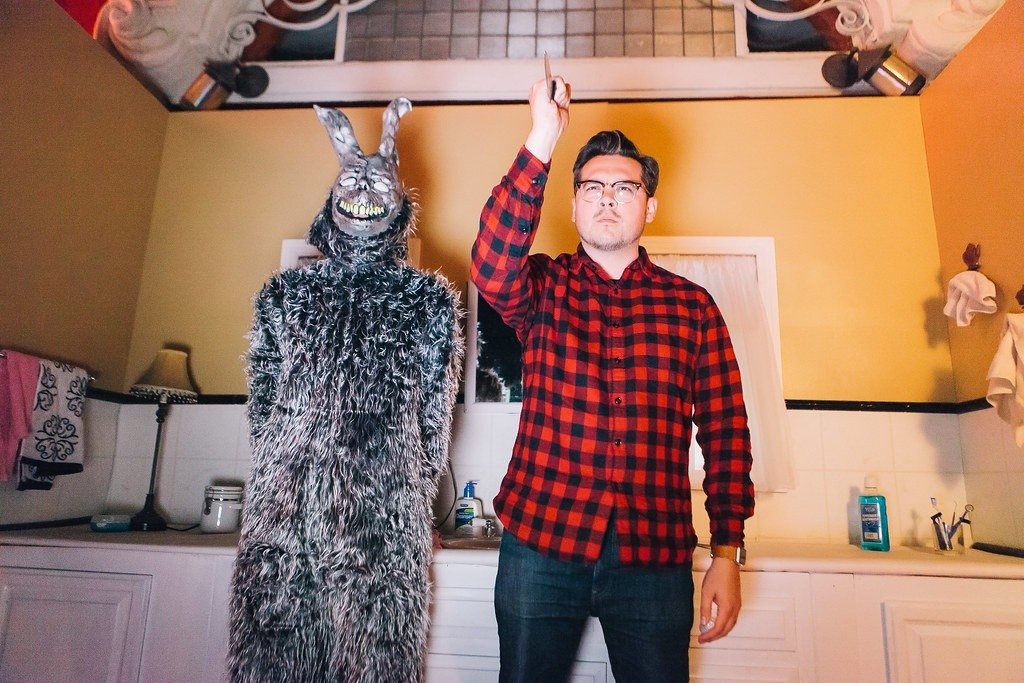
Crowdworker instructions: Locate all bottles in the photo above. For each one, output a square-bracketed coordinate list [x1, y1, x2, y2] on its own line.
[199, 485, 243, 534]
[857, 476, 891, 552]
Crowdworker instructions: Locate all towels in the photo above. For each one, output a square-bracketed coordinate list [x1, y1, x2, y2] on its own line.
[0, 349, 89, 492]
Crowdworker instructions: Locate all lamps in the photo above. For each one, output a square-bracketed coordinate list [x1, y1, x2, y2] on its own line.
[180, 58, 268, 112]
[128, 348, 198, 532]
[821, 46, 927, 96]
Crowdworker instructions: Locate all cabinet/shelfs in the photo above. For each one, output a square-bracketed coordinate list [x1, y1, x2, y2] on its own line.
[420, 586, 1024, 683]
[0, 565, 153, 683]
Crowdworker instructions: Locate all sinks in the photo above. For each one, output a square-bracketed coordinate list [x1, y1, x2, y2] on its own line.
[441, 536, 500, 552]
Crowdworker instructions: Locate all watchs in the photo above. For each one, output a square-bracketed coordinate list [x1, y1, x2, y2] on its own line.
[709, 545, 746, 566]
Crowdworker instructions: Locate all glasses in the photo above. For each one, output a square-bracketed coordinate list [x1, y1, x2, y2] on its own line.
[574, 180, 651, 204]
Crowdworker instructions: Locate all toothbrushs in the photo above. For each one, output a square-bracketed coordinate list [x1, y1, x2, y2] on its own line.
[929, 497, 950, 551]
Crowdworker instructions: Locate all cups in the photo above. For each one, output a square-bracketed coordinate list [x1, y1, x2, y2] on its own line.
[931, 524, 959, 555]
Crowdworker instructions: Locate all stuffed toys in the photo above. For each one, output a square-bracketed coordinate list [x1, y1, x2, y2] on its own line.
[226, 97, 464, 683]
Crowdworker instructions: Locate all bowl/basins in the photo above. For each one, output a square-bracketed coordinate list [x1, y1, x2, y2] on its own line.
[89, 514, 131, 531]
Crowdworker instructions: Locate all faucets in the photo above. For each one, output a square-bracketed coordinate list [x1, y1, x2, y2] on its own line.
[484, 518, 496, 539]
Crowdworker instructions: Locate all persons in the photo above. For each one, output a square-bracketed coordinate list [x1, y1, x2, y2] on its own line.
[471, 76, 756, 683]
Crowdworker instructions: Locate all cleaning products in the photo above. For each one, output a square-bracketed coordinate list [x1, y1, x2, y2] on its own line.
[454, 481, 483, 536]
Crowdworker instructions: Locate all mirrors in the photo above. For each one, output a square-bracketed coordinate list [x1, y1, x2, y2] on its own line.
[464, 280, 522, 413]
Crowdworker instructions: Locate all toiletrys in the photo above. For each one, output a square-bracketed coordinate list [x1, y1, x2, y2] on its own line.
[857, 476, 891, 551]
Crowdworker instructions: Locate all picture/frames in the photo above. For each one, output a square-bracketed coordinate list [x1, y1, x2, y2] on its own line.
[281, 237, 421, 270]
